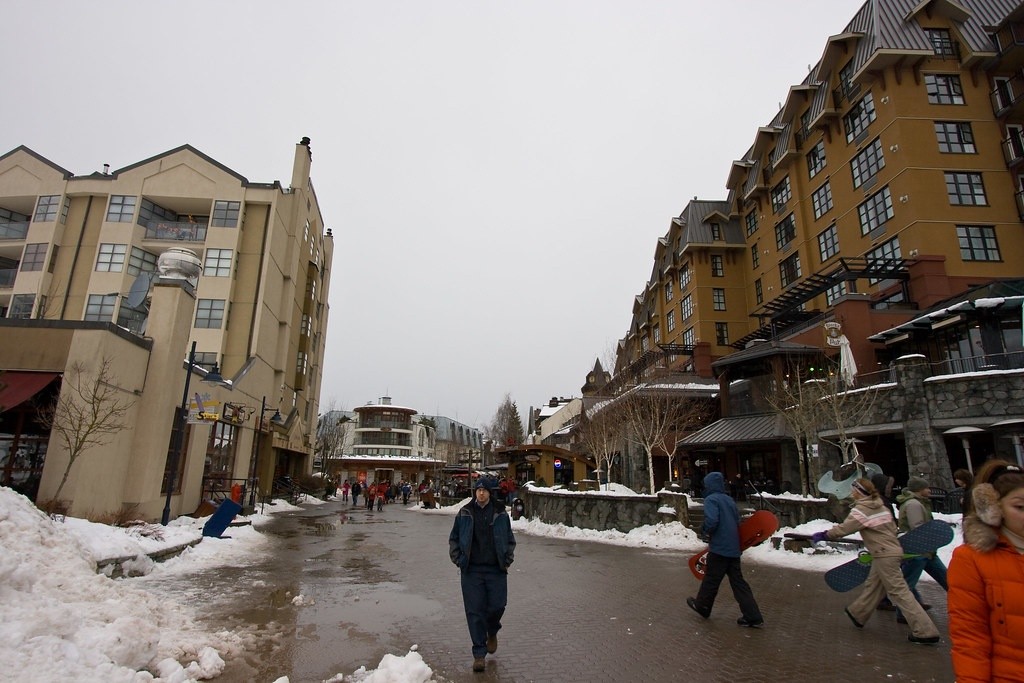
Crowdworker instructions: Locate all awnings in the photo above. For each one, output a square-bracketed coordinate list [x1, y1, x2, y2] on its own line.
[0, 371, 59, 414]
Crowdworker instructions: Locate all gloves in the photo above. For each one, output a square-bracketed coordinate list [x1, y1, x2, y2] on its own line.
[812, 530, 830, 544]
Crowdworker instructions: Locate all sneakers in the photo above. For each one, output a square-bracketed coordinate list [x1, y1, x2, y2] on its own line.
[486, 635, 497, 654]
[737, 616, 764, 627]
[472, 656, 486, 671]
[686, 597, 710, 619]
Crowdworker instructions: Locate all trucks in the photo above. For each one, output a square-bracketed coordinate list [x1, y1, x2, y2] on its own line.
[452, 473, 481, 500]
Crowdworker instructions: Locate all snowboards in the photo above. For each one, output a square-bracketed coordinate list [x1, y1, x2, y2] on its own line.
[688, 509, 780, 579]
[824, 520, 953, 591]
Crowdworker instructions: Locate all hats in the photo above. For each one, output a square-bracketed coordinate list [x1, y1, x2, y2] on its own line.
[907, 477, 929, 492]
[475, 478, 491, 492]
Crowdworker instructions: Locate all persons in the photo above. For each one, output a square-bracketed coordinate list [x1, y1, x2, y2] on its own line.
[687, 472, 764, 627]
[322, 476, 516, 512]
[449, 478, 517, 672]
[812, 457, 1024, 683]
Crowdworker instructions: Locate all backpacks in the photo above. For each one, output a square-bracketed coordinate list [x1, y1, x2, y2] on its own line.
[370, 487, 375, 495]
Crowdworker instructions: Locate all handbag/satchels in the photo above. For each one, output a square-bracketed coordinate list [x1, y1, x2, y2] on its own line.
[341, 487, 346, 491]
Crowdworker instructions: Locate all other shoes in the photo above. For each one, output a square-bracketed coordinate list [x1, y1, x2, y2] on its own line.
[846, 608, 864, 630]
[876, 605, 896, 611]
[921, 604, 931, 611]
[907, 633, 939, 643]
[896, 616, 908, 624]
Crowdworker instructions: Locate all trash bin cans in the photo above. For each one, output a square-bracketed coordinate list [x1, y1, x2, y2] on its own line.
[511, 497, 524, 520]
[420, 489, 436, 508]
[202, 498, 242, 538]
